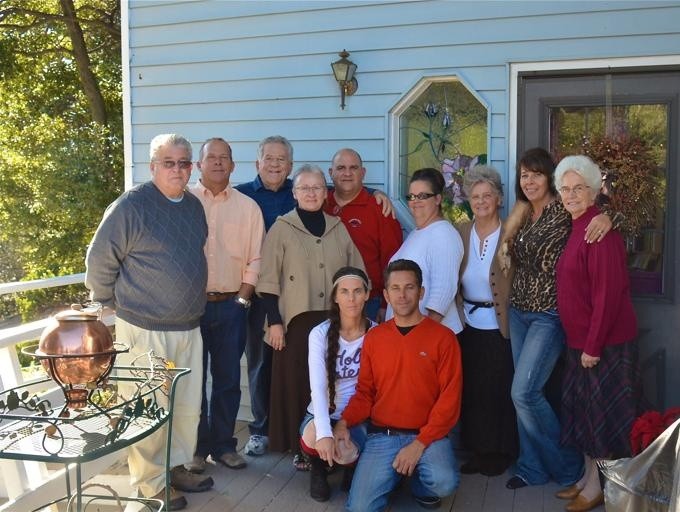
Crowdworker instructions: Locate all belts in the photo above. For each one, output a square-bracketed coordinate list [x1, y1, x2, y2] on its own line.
[207, 292, 236, 302]
[462, 297, 495, 314]
[367, 426, 449, 438]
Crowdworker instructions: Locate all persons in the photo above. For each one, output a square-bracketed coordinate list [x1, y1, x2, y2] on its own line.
[184, 138, 267, 473]
[553, 155, 637, 511]
[321, 148, 402, 324]
[232, 136, 398, 455]
[385, 167, 465, 354]
[299, 264, 380, 500]
[460, 164, 508, 476]
[333, 259, 463, 511]
[85, 133, 214, 512]
[255, 163, 372, 469]
[488, 148, 614, 490]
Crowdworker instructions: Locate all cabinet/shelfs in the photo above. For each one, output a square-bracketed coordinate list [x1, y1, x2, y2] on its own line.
[0, 367, 192, 512]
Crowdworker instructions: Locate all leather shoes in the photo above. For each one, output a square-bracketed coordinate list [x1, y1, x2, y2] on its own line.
[507, 475, 527, 489]
[555, 484, 581, 499]
[565, 492, 604, 512]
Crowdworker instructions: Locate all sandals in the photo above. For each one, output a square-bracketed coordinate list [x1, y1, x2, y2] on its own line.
[294, 454, 312, 471]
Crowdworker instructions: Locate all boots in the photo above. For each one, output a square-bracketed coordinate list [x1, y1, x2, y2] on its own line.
[310, 457, 331, 502]
[341, 468, 355, 491]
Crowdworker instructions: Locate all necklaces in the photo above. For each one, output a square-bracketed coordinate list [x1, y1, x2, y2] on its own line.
[475, 224, 500, 248]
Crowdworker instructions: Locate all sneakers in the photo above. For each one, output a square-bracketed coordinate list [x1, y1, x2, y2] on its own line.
[138, 485, 187, 512]
[245, 435, 268, 456]
[211, 452, 247, 470]
[415, 496, 441, 509]
[183, 456, 206, 474]
[170, 465, 214, 492]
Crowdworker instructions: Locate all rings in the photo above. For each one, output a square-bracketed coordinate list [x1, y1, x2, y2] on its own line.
[599, 230, 603, 234]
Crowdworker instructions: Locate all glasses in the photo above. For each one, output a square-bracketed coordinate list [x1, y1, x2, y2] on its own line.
[163, 160, 191, 169]
[561, 185, 590, 193]
[300, 184, 322, 194]
[406, 192, 437, 201]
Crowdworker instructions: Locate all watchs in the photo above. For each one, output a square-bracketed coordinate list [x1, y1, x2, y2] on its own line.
[235, 298, 251, 309]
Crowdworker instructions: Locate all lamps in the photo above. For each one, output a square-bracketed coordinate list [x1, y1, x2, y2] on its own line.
[331, 49, 358, 111]
